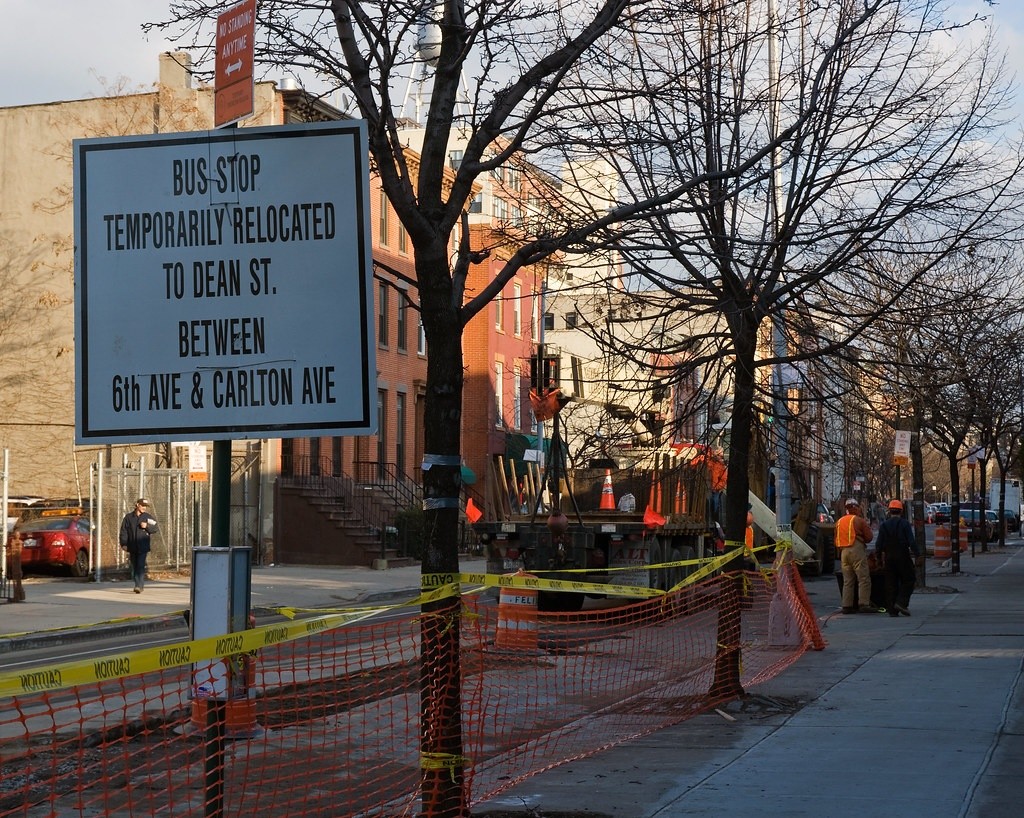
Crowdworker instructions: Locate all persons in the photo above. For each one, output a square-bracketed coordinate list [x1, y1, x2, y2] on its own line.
[740, 512, 755, 609]
[834, 498, 877, 614]
[120, 499, 159, 593]
[875, 500, 919, 617]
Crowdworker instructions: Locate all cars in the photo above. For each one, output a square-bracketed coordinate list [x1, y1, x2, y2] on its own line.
[817, 502, 834, 525]
[1006, 509, 1016, 532]
[986, 510, 1000, 541]
[935, 507, 952, 525]
[6, 516, 96, 577]
[910, 500, 948, 524]
[959, 510, 994, 543]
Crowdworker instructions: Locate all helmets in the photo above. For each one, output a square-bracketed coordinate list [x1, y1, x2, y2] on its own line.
[845, 499, 860, 506]
[746, 511, 753, 523]
[888, 499, 903, 509]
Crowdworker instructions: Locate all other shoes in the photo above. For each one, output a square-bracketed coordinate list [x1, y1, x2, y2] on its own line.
[134, 586, 144, 594]
[888, 610, 899, 617]
[740, 603, 752, 611]
[842, 606, 857, 614]
[860, 605, 878, 613]
[894, 602, 911, 616]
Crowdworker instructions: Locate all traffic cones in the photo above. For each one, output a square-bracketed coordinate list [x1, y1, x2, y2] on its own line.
[648, 469, 661, 514]
[594, 468, 619, 510]
[675, 477, 685, 514]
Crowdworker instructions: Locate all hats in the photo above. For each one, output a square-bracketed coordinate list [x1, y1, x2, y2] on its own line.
[138, 499, 150, 506]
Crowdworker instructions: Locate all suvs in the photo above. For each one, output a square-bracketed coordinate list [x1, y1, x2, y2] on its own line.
[1, 495, 47, 535]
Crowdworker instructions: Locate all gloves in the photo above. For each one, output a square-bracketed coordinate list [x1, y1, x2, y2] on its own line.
[915, 557, 923, 568]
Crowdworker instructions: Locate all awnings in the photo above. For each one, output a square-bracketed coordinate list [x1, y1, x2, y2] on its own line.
[460, 465, 476, 485]
[504, 433, 566, 480]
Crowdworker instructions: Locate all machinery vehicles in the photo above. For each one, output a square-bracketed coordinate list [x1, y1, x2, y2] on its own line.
[473, 389, 726, 618]
[748, 424, 841, 579]
